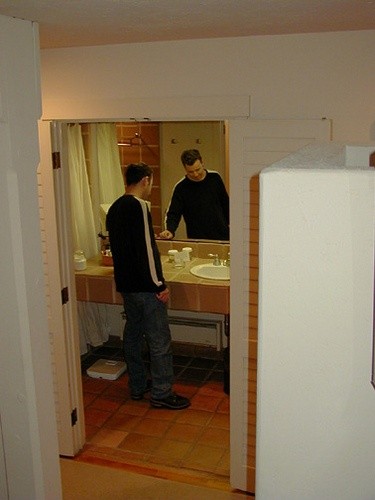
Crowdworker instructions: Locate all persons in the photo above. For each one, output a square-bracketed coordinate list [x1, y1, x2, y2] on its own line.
[158, 149, 229, 240]
[106, 162, 191, 409]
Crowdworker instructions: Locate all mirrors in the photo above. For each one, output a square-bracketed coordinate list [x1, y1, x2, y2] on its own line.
[66, 120, 230, 243]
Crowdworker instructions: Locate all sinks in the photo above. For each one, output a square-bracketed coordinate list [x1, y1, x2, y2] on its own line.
[190, 264, 230, 281]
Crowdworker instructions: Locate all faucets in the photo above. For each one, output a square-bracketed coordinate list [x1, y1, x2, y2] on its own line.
[223, 252, 230, 266]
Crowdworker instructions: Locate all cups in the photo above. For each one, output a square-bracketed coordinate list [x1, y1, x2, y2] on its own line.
[102, 250, 114, 266]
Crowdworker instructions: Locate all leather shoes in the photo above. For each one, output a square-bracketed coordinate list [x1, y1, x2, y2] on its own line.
[150, 395, 190, 409]
[131, 394, 144, 400]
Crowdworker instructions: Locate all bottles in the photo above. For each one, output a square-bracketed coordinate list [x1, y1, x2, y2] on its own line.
[73, 251, 88, 270]
[168, 250, 178, 263]
[182, 247, 192, 261]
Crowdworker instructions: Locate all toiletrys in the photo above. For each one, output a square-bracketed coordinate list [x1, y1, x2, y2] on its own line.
[72, 249, 87, 271]
